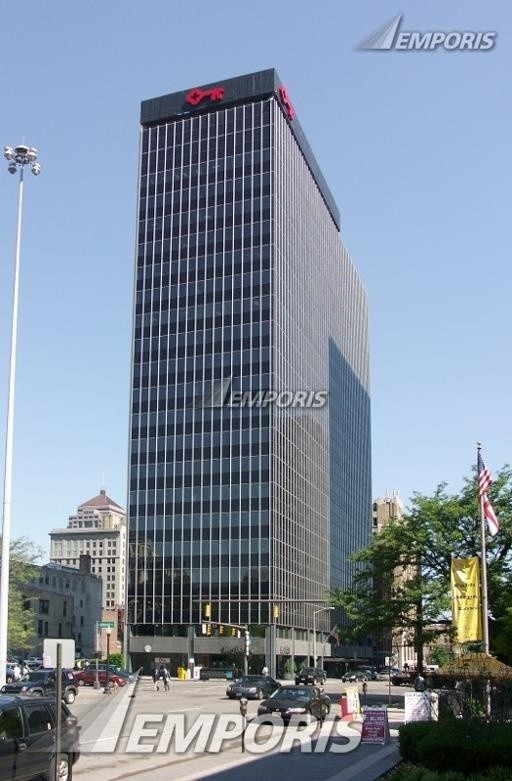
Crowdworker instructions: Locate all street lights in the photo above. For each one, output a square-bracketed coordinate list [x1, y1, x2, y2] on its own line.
[103, 629, 112, 696]
[313, 606, 335, 669]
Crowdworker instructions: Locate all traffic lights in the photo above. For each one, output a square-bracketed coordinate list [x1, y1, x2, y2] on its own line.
[202, 603, 236, 637]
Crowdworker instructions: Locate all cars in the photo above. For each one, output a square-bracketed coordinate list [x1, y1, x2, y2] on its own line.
[73, 671, 127, 687]
[342, 664, 440, 686]
[226, 675, 281, 699]
[85, 664, 138, 684]
[0, 655, 79, 705]
[257, 686, 331, 727]
[295, 667, 327, 686]
[0, 695, 82, 781]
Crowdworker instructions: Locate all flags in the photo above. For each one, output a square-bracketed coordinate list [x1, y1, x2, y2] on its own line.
[331, 627, 339, 648]
[476, 452, 501, 541]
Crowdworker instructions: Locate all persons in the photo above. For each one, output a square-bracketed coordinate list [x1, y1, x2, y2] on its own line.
[76, 659, 83, 672]
[83, 660, 90, 670]
[260, 663, 269, 677]
[160, 668, 171, 691]
[239, 667, 245, 679]
[152, 669, 161, 692]
[233, 667, 239, 683]
[415, 672, 425, 692]
